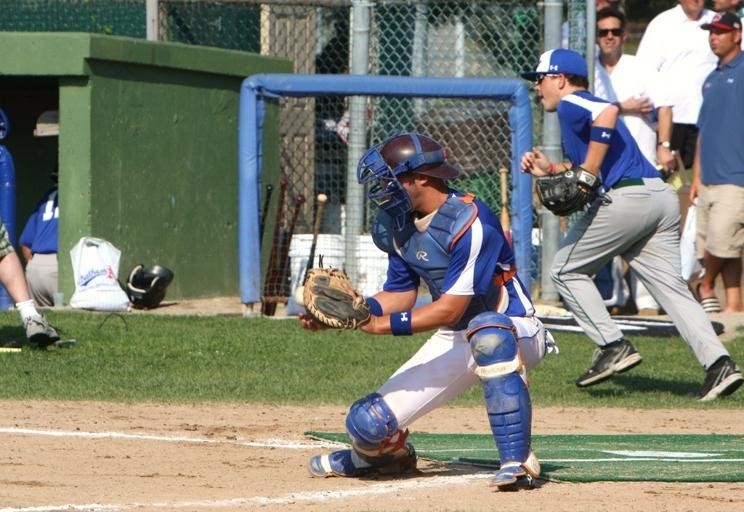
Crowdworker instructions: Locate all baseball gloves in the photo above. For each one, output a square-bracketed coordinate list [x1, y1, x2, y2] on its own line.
[535, 164, 601, 217]
[301, 266, 374, 331]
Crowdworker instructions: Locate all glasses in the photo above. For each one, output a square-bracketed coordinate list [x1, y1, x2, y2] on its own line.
[534, 73, 560, 83]
[596, 28, 625, 39]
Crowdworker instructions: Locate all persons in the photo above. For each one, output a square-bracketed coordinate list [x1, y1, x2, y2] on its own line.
[0, 217, 59, 346]
[295, 132, 557, 490]
[18, 186, 59, 309]
[690, 12, 744, 313]
[631, 1, 716, 172]
[712, 0, 744, 52]
[518, 49, 744, 405]
[591, 10, 673, 317]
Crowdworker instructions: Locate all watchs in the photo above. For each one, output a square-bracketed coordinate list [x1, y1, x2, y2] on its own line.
[655, 142, 672, 150]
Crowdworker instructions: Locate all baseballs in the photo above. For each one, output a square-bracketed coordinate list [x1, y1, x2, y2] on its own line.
[294, 285, 306, 307]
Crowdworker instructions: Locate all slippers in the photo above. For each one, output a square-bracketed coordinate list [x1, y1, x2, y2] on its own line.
[694, 281, 723, 314]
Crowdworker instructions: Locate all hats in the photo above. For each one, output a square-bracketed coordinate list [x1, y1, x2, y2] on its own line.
[378, 135, 461, 181]
[700, 10, 743, 33]
[519, 47, 589, 83]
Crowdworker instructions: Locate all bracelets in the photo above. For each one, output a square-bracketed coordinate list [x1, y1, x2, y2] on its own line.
[388, 310, 412, 338]
[363, 295, 383, 318]
[612, 103, 622, 115]
[589, 125, 614, 145]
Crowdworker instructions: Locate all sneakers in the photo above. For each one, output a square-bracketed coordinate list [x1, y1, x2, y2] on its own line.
[575, 338, 644, 387]
[308, 442, 417, 478]
[695, 359, 744, 403]
[22, 315, 60, 345]
[488, 449, 543, 490]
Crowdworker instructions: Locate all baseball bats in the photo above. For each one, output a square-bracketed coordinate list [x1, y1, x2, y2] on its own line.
[499, 167, 512, 247]
[303, 194, 328, 287]
[261, 180, 305, 315]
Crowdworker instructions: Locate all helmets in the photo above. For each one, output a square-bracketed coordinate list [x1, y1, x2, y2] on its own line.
[126, 264, 174, 309]
